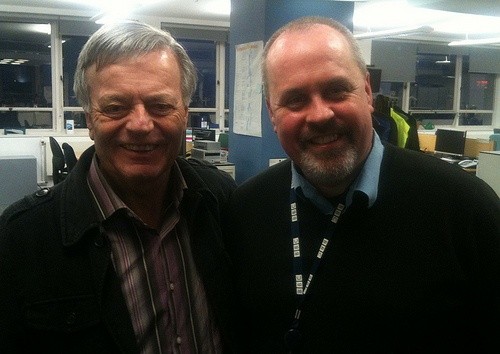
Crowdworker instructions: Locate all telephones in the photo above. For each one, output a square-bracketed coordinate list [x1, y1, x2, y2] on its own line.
[459, 159, 478, 169]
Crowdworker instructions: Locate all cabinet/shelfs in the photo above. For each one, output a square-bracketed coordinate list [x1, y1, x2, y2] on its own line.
[477, 151, 500, 198]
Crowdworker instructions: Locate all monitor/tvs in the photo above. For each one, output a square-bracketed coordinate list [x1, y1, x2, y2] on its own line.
[434, 129, 466, 156]
[192, 129, 215, 141]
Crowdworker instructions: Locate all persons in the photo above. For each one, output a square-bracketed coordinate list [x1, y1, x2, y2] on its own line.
[0, 23, 238, 354]
[223, 17, 500, 354]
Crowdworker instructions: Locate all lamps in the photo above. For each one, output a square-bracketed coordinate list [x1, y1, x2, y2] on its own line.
[449, 34, 500, 46]
[354, 26, 433, 40]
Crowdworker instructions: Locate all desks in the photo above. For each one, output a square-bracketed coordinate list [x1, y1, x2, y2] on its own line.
[418, 133, 495, 171]
[214, 162, 236, 182]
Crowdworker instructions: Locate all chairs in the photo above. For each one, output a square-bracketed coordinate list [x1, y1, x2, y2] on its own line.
[50, 136, 78, 186]
